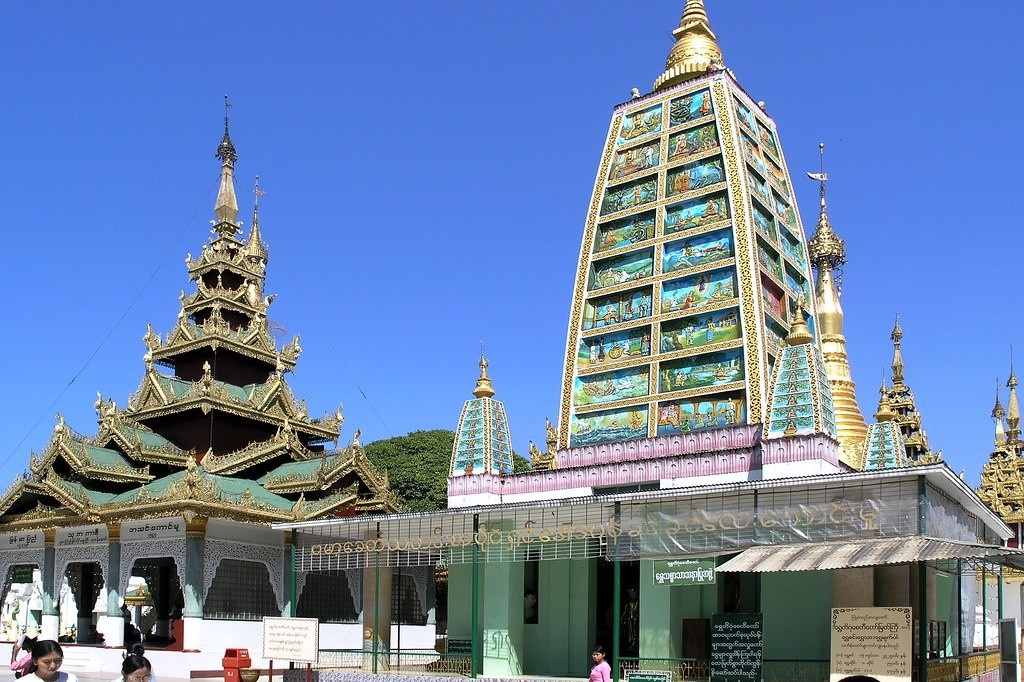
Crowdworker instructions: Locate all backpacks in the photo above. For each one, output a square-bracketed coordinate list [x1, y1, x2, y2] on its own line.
[29, 662, 38, 673]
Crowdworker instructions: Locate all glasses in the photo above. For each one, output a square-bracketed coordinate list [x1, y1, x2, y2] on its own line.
[125, 675, 152, 682]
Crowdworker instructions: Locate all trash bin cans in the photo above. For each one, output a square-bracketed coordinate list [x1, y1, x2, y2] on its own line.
[221, 647, 252, 682]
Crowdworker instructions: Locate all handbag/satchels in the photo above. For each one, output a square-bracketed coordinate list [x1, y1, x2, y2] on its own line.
[15, 672, 21, 678]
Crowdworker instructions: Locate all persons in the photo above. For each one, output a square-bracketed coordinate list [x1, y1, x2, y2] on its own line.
[129, 624, 141, 644]
[87, 624, 99, 644]
[108, 643, 158, 682]
[589, 646, 611, 682]
[9, 632, 79, 682]
